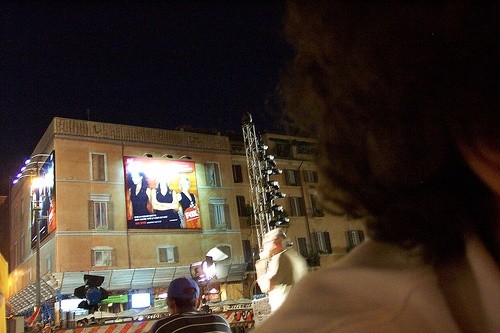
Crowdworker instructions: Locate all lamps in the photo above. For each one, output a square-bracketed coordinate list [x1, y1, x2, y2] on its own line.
[257, 142, 290, 227]
[189, 247, 229, 304]
[141, 153, 192, 160]
[12, 154, 50, 185]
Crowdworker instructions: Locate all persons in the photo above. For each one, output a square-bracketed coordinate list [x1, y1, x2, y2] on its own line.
[247, 1, 500, 333]
[126, 169, 198, 229]
[147, 276, 232, 333]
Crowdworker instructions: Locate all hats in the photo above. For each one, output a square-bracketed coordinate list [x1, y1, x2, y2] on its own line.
[168, 276, 199, 298]
[43, 324, 52, 329]
[35, 323, 42, 327]
